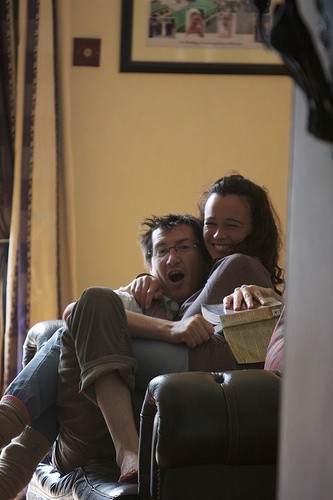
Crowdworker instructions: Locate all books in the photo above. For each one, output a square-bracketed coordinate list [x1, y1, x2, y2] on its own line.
[200, 296, 283, 363]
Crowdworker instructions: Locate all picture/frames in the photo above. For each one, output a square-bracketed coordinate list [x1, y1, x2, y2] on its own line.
[119, 0, 290, 76]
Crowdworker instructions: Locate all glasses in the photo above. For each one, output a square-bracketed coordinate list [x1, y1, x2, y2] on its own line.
[148, 242, 199, 257]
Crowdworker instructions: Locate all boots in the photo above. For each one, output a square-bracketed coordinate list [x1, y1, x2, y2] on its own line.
[0, 395, 30, 450]
[0, 425, 51, 500]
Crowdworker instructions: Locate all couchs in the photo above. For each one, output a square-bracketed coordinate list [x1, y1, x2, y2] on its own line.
[22, 320, 279, 500]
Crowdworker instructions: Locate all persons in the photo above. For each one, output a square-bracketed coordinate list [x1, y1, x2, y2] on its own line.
[0, 212, 283, 485]
[0, 173, 285, 500]
